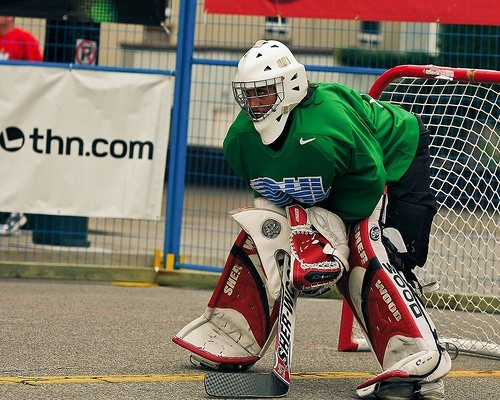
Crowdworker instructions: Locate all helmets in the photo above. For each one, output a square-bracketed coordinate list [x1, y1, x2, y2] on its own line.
[231, 40, 309, 145]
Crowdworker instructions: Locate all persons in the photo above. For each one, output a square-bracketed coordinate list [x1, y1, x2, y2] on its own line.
[0, 16, 42, 234]
[171, 40, 452, 400]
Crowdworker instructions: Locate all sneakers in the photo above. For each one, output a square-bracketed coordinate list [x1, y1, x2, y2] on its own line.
[0, 214, 27, 234]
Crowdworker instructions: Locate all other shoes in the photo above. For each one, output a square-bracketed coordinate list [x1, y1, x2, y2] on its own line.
[375, 383, 416, 399]
[200, 360, 241, 373]
